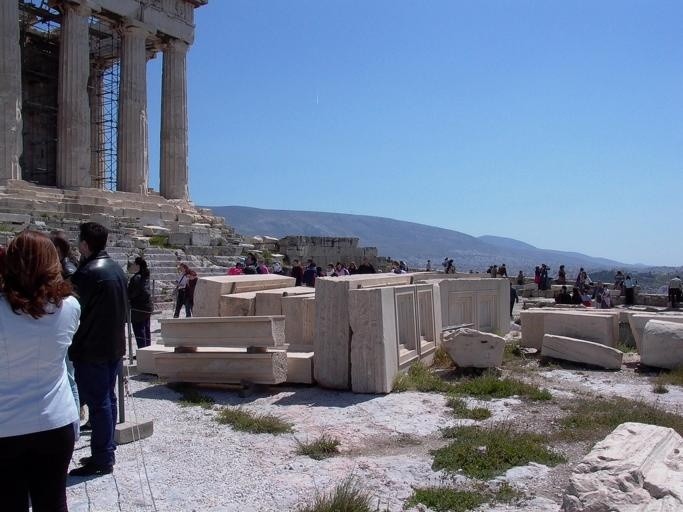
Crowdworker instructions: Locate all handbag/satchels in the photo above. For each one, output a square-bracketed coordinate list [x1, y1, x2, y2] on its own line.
[171, 288, 178, 299]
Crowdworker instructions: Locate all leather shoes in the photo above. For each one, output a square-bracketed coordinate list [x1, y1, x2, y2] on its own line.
[79, 454, 115, 466]
[80, 419, 92, 431]
[70, 460, 113, 476]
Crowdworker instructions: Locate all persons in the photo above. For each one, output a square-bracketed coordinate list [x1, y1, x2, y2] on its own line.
[426, 257, 453, 274]
[174, 263, 197, 317]
[230, 251, 375, 287]
[487, 264, 634, 317]
[128, 258, 152, 359]
[387, 255, 408, 274]
[668, 275, 683, 309]
[1, 222, 129, 512]
